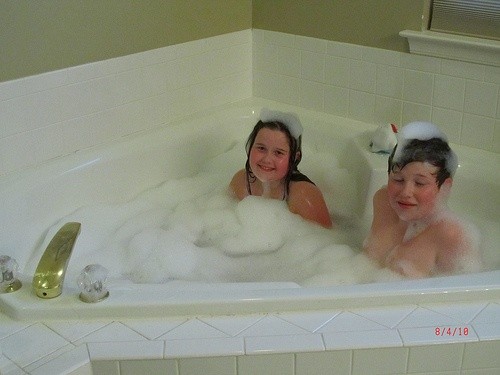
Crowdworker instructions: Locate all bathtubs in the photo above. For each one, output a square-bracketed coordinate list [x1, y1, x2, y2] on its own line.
[0, 96, 499, 322]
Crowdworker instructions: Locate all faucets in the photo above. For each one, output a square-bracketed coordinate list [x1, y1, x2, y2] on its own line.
[31, 221, 82, 299]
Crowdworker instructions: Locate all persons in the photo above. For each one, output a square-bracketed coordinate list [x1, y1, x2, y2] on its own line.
[229, 119, 333, 229]
[361, 139, 481, 281]
